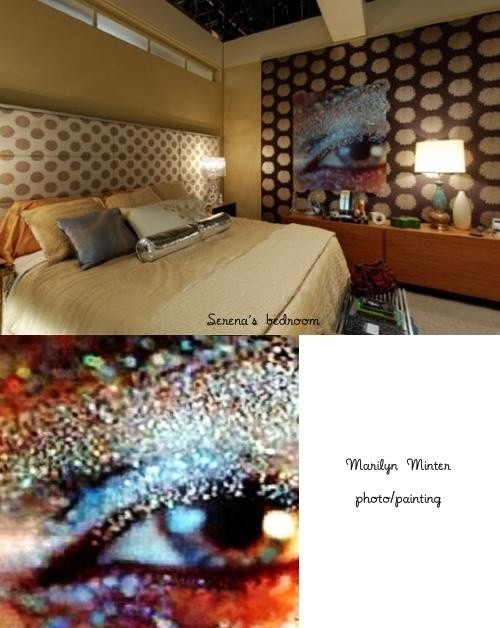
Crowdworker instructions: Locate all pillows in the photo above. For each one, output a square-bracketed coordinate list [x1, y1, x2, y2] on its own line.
[1, 183, 204, 276]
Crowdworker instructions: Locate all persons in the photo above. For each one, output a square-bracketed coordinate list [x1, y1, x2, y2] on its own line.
[0, 335, 299, 628]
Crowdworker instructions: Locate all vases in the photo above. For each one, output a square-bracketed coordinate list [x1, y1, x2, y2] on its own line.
[450, 189, 472, 229]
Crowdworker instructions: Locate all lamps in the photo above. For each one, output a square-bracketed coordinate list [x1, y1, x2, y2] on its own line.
[413, 138, 466, 229]
[202, 156, 229, 213]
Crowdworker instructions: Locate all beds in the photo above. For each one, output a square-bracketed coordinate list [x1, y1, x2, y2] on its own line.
[1, 181, 353, 336]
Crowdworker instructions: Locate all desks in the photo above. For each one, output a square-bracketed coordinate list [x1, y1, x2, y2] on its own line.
[280, 208, 500, 308]
